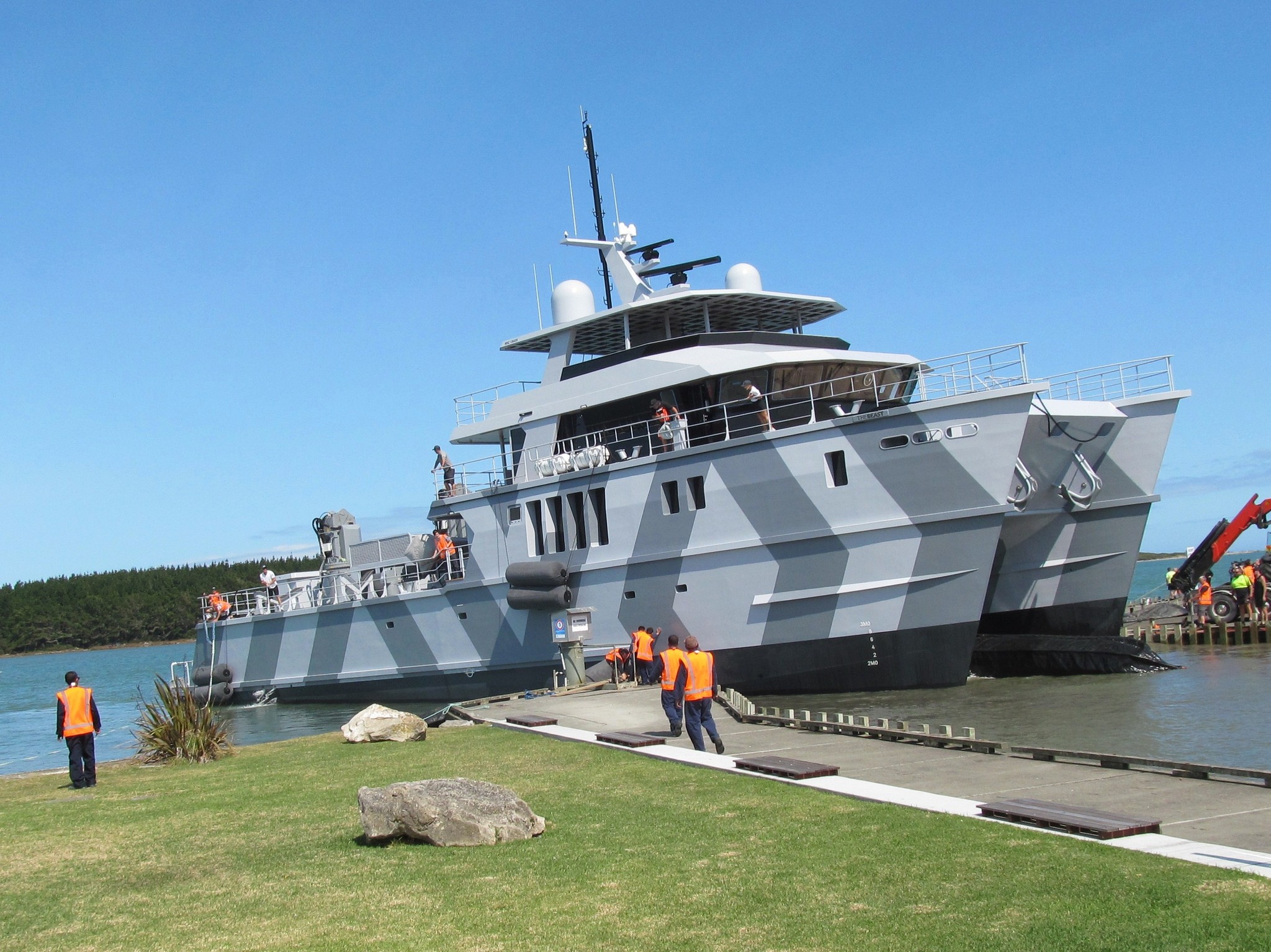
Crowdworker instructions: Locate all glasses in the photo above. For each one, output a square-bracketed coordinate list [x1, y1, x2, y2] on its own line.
[77, 677, 80, 680]
[263, 567, 267, 569]
[1235, 571, 1239, 573]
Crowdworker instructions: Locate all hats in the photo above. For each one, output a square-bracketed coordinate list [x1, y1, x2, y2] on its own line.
[212, 587, 216, 590]
[1251, 566, 1260, 570]
[261, 565, 266, 569]
[684, 636, 698, 649]
[433, 446, 440, 451]
[742, 380, 752, 387]
[651, 399, 661, 408]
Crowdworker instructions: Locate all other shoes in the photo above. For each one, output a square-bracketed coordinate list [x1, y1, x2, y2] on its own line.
[459, 573, 463, 578]
[768, 427, 776, 431]
[643, 681, 650, 688]
[452, 575, 457, 579]
[448, 493, 455, 497]
[280, 607, 284, 611]
[714, 738, 725, 754]
[86, 783, 97, 787]
[68, 784, 86, 790]
[1241, 622, 1245, 626]
[1197, 623, 1204, 628]
[1258, 621, 1265, 626]
[670, 721, 683, 737]
[611, 677, 615, 683]
[649, 680, 658, 685]
[762, 430, 767, 433]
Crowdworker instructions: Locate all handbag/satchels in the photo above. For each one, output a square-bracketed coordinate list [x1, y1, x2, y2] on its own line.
[657, 418, 673, 439]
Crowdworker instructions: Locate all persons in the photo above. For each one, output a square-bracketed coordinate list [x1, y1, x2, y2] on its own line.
[1173, 568, 1182, 598]
[431, 445, 455, 497]
[636, 627, 657, 686]
[259, 565, 283, 610]
[203, 587, 221, 613]
[673, 636, 725, 755]
[649, 635, 689, 737]
[741, 380, 776, 433]
[650, 399, 680, 452]
[629, 626, 662, 682]
[1188, 576, 1220, 627]
[1229, 554, 1271, 627]
[1165, 568, 1176, 599]
[1206, 569, 1213, 583]
[431, 530, 461, 579]
[208, 599, 240, 622]
[605, 648, 629, 683]
[56, 671, 101, 788]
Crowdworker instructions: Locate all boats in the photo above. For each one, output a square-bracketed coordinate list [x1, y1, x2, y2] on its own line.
[170, 99, 1193, 714]
